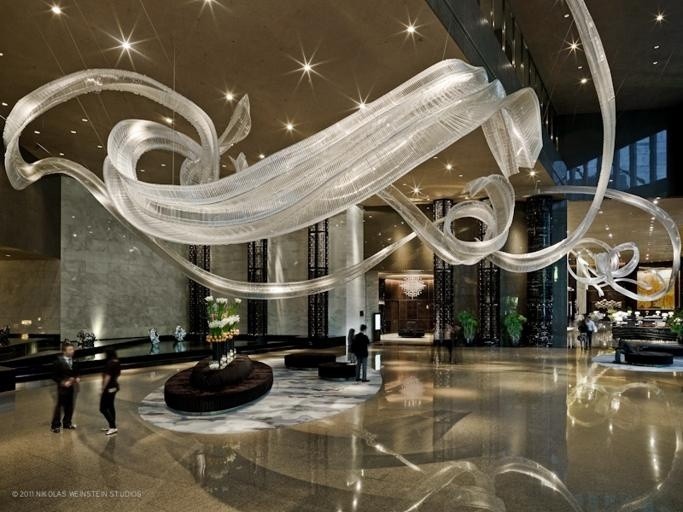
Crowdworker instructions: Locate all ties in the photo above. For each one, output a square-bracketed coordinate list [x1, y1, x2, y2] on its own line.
[68, 359, 72, 369]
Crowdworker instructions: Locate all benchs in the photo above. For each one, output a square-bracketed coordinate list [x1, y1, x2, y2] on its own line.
[284, 351, 357, 381]
[629, 351, 673, 366]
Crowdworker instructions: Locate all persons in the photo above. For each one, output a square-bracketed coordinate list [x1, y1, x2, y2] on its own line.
[595, 307, 606, 321]
[0, 325, 9, 342]
[584, 316, 597, 351]
[51, 343, 81, 433]
[429, 320, 466, 365]
[586, 349, 593, 384]
[577, 319, 589, 350]
[578, 350, 587, 379]
[347, 328, 357, 363]
[351, 324, 371, 382]
[46, 426, 80, 493]
[98, 346, 121, 435]
[98, 432, 123, 510]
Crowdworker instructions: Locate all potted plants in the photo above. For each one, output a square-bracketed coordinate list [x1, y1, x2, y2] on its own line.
[504, 312, 527, 346]
[458, 310, 479, 346]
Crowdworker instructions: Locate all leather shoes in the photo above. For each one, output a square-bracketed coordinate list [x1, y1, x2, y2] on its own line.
[63, 424, 76, 430]
[51, 426, 60, 433]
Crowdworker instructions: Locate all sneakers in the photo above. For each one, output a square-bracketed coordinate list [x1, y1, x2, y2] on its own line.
[362, 378, 370, 382]
[101, 426, 109, 431]
[105, 428, 118, 435]
[356, 378, 362, 381]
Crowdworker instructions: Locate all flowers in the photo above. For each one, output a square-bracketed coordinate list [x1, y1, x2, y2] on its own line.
[203, 295, 241, 342]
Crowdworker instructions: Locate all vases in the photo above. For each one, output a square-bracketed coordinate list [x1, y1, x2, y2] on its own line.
[206, 338, 237, 370]
